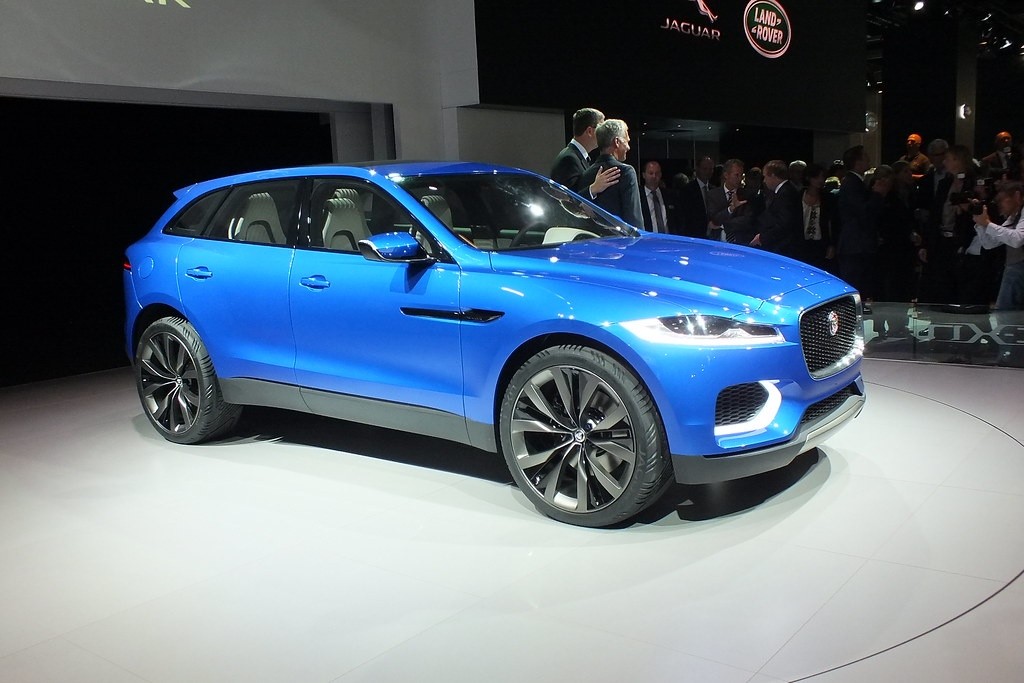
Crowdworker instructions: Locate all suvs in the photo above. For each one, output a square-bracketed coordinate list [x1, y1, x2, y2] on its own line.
[117, 158, 867, 527]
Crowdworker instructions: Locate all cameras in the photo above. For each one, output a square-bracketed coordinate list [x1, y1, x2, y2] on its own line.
[971, 178, 999, 217]
[948, 173, 975, 206]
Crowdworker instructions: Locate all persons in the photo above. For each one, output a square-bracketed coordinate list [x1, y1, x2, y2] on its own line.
[550, 108, 621, 201]
[578, 119, 645, 231]
[640, 132, 1024, 310]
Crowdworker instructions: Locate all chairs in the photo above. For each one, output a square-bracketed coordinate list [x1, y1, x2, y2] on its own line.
[414, 195, 455, 251]
[321, 188, 373, 250]
[227, 191, 288, 245]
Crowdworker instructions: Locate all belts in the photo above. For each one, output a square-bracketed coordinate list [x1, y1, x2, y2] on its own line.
[937, 230, 956, 239]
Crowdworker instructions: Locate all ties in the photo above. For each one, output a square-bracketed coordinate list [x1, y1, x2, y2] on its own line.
[726, 191, 736, 244]
[807, 207, 818, 239]
[702, 185, 709, 214]
[650, 190, 665, 234]
[1003, 154, 1010, 169]
